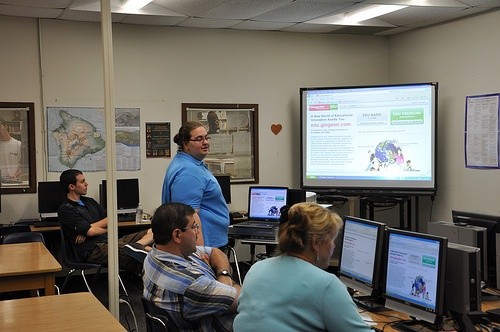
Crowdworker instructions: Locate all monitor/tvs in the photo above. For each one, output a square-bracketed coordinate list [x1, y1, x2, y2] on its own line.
[300, 81, 438, 194]
[214, 175, 231, 204]
[38, 181, 70, 218]
[337, 214, 447, 332]
[101, 178, 140, 214]
[451, 209, 500, 299]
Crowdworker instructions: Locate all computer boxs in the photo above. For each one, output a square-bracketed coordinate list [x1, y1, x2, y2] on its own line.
[426, 220, 489, 314]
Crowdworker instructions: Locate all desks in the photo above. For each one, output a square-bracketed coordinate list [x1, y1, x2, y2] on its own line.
[0, 242, 62, 296]
[0, 292, 128, 332]
[357, 292, 500, 332]
[30, 216, 152, 232]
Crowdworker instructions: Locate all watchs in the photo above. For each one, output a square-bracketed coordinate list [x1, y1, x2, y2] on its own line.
[216, 269, 233, 280]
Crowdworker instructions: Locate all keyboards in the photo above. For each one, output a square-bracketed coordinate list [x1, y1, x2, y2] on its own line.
[34, 220, 61, 227]
[118, 215, 137, 222]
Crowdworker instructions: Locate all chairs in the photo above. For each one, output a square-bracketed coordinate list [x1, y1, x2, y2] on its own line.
[5, 231, 243, 332]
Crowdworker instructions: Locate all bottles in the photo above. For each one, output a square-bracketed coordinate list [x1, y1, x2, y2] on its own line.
[136, 203, 143, 224]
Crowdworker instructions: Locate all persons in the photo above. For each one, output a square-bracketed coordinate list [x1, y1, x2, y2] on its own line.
[58, 170, 155, 273]
[0, 118, 24, 180]
[142, 121, 242, 332]
[233, 202, 375, 332]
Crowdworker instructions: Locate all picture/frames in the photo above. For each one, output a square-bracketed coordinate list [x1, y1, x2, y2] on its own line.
[0, 102, 37, 194]
[181, 102, 260, 184]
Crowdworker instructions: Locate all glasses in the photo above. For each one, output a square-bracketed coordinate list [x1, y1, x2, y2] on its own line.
[188, 135, 211, 143]
[181, 223, 199, 231]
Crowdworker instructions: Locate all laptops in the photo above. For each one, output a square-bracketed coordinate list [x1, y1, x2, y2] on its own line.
[233, 187, 289, 229]
